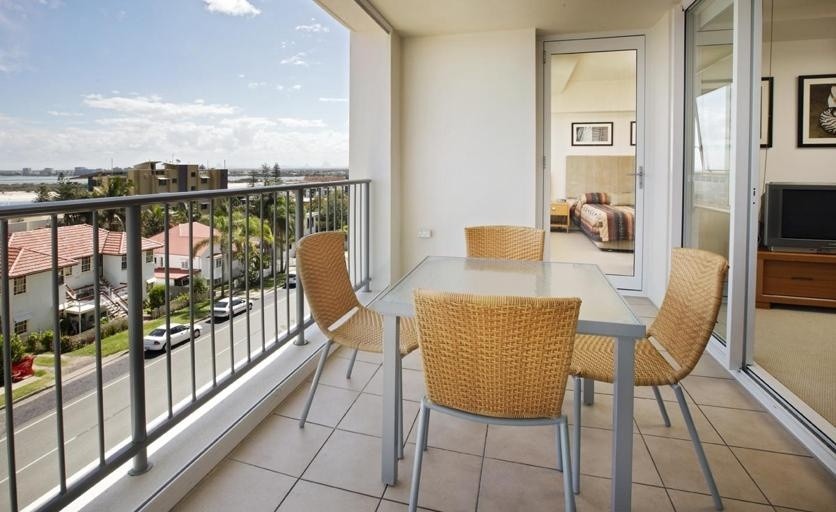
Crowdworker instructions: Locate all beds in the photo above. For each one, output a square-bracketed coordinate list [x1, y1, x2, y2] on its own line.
[566, 155, 634, 252]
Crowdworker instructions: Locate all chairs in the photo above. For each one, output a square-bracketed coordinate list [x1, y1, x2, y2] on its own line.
[568, 248, 729, 511]
[408, 288, 583, 512]
[295, 230, 419, 459]
[465, 224, 544, 262]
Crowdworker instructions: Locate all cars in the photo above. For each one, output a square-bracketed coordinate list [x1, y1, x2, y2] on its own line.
[143, 325, 202, 353]
[209, 296, 253, 321]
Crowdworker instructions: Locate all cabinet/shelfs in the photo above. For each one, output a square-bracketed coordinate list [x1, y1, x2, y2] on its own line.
[757, 251, 835, 306]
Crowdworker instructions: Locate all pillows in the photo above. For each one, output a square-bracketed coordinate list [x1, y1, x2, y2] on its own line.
[608, 193, 635, 205]
[579, 192, 607, 204]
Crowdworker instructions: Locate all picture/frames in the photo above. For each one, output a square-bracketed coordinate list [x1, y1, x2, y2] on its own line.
[630, 121, 636, 146]
[797, 75, 836, 146]
[760, 77, 774, 147]
[571, 122, 613, 146]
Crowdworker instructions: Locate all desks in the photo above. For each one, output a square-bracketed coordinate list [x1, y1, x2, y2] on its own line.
[364, 254, 646, 512]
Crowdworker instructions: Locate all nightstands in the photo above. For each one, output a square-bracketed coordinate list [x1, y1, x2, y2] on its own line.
[550, 203, 569, 233]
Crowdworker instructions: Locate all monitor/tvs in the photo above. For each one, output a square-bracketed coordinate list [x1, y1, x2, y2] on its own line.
[761, 181, 835, 254]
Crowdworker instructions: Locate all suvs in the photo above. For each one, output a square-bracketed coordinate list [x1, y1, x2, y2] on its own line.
[284, 271, 299, 288]
